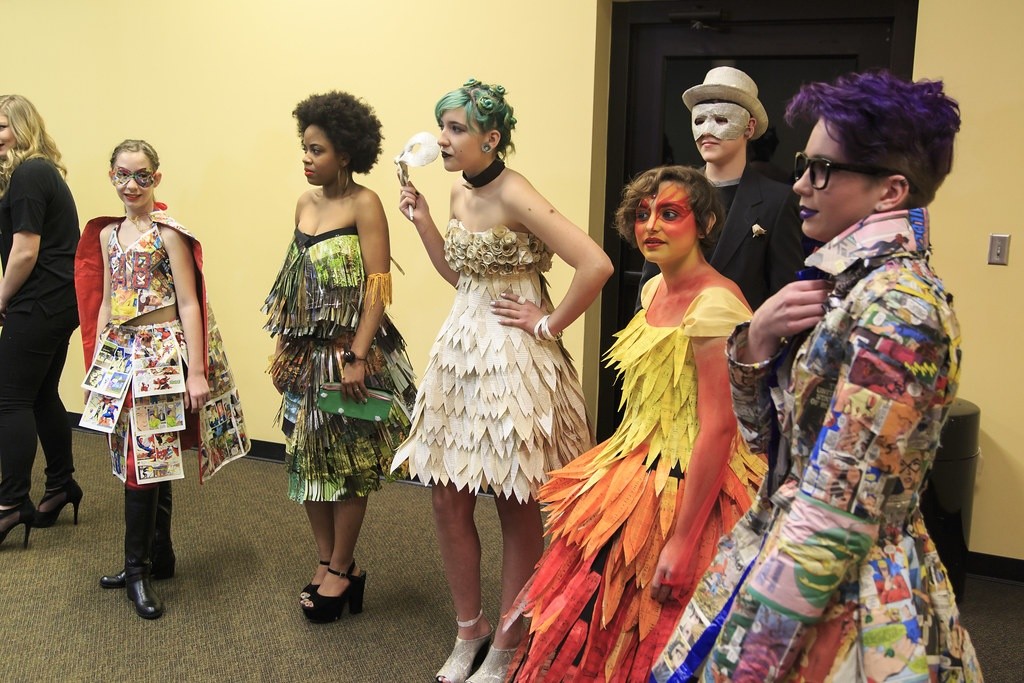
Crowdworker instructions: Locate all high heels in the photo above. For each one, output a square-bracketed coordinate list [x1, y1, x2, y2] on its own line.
[300, 559, 367, 622]
[37, 480, 84, 527]
[461, 642, 526, 683]
[0, 499, 37, 549]
[298, 562, 331, 598]
[436, 609, 492, 683]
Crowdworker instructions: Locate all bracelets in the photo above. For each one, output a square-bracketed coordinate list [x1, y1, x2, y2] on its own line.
[534, 315, 564, 341]
[344, 351, 366, 363]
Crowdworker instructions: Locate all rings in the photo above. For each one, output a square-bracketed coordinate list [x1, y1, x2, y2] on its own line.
[354, 389, 359, 391]
[517, 296, 526, 304]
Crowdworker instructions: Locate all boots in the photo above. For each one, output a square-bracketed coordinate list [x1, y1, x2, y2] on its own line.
[101, 542, 176, 588]
[125, 564, 163, 620]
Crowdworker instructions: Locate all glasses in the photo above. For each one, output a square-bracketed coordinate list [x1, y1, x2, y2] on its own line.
[793, 151, 919, 200]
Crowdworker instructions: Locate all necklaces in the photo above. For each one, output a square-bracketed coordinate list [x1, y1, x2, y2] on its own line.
[462, 154, 505, 188]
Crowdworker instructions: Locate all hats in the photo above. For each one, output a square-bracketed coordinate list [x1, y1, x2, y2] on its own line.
[681, 66, 768, 139]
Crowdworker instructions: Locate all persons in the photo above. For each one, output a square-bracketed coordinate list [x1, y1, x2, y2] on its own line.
[389, 79, 614, 683]
[0, 94, 84, 548]
[647, 72, 983, 683]
[262, 92, 420, 626]
[73, 139, 252, 618]
[500, 166, 771, 683]
[635, 67, 805, 314]
